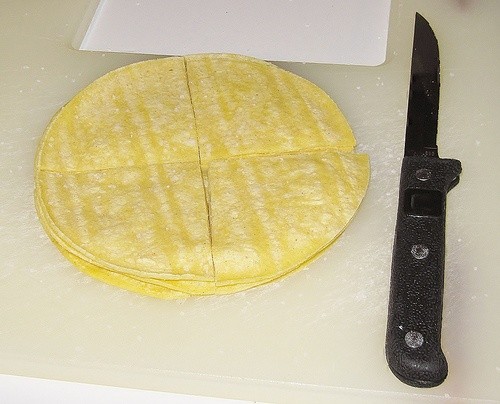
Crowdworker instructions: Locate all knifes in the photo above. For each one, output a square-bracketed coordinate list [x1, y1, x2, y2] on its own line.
[384, 11, 463, 387]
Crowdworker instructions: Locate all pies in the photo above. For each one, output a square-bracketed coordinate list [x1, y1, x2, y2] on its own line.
[33, 52, 372, 300]
